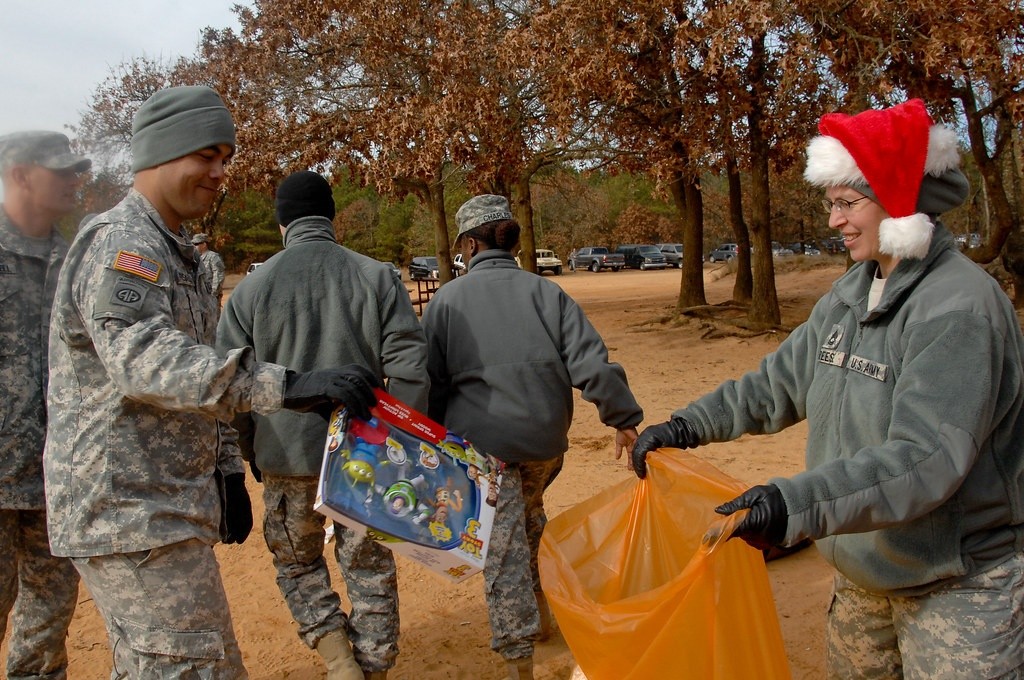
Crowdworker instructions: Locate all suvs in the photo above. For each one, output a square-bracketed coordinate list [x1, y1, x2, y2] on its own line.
[655, 244, 705, 268]
[515, 248, 564, 276]
[409, 257, 459, 278]
[709, 243, 754, 264]
[615, 243, 668, 270]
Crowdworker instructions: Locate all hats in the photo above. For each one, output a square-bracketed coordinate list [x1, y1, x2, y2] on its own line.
[854, 168, 970, 221]
[1, 131, 92, 172]
[275, 171, 335, 227]
[191, 234, 208, 244]
[452, 194, 513, 250]
[803, 98, 961, 261]
[130, 86, 235, 174]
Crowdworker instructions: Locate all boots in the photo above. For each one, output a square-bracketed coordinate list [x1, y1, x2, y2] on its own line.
[316, 631, 363, 679]
[502, 658, 534, 680]
[532, 592, 550, 642]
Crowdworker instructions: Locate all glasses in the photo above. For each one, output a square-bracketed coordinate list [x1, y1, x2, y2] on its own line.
[821, 195, 869, 215]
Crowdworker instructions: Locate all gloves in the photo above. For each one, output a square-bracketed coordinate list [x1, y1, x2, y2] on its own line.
[283, 364, 380, 424]
[632, 416, 699, 479]
[701, 483, 788, 549]
[221, 473, 253, 545]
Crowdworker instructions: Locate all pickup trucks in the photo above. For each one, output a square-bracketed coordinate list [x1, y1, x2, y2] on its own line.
[567, 245, 628, 271]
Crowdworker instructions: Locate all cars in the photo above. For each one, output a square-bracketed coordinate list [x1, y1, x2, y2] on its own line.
[792, 243, 821, 255]
[771, 242, 794, 257]
[452, 253, 467, 276]
[382, 262, 406, 279]
[955, 232, 983, 249]
[246, 263, 263, 276]
[805, 236, 846, 253]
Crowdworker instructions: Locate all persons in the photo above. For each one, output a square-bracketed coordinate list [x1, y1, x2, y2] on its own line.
[632, 98, 1024, 680]
[217, 170, 426, 680]
[191, 234, 224, 324]
[41, 85, 379, 680]
[422, 194, 643, 680]
[0, 131, 94, 680]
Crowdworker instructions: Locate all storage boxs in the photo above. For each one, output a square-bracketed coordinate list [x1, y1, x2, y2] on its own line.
[314, 388, 507, 584]
